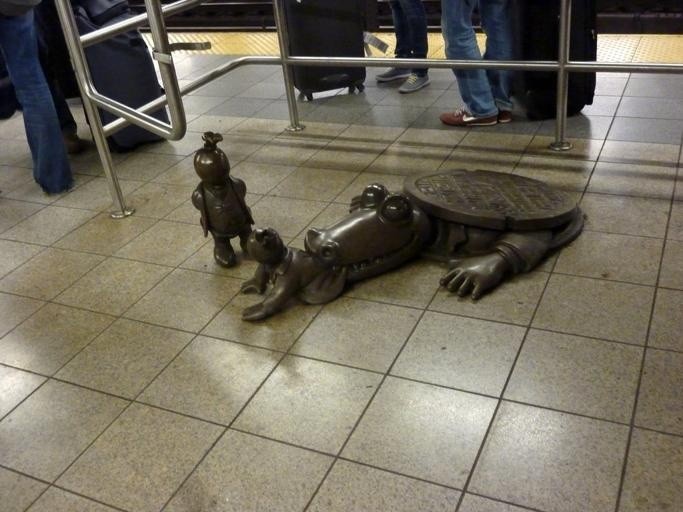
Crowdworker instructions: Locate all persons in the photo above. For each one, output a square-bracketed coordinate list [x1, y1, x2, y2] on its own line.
[374, 1, 431, 95]
[1, 0, 84, 194]
[436, 1, 516, 127]
[238, 229, 347, 322]
[190, 131, 257, 267]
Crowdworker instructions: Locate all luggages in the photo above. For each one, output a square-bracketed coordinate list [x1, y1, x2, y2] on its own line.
[286, 0, 367, 93]
[511, 0, 598, 118]
[73, 0, 174, 154]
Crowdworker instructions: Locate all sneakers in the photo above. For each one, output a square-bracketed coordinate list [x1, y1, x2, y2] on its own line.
[398, 72, 430, 94]
[63, 127, 79, 152]
[496, 108, 512, 123]
[374, 66, 411, 81]
[439, 106, 499, 126]
